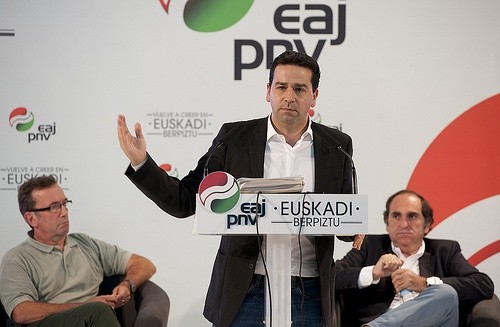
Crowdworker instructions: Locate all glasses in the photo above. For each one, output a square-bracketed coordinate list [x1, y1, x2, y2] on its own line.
[31, 200, 72, 212]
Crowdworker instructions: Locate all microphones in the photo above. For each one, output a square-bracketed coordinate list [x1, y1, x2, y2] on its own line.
[203, 140, 225, 178]
[311, 122, 358, 194]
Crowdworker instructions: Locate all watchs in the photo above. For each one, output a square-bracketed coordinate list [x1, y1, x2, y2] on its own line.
[124, 279, 136, 294]
[425, 277, 436, 287]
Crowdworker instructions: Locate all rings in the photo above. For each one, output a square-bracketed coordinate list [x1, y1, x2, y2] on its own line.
[391, 257, 394, 261]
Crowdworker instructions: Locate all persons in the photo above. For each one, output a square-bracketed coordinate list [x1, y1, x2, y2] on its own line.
[0, 175, 156, 327]
[117, 51, 358, 327]
[334, 190, 494, 327]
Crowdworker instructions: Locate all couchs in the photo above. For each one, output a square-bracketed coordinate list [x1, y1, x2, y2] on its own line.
[461, 294, 500, 327]
[0, 275, 170, 327]
